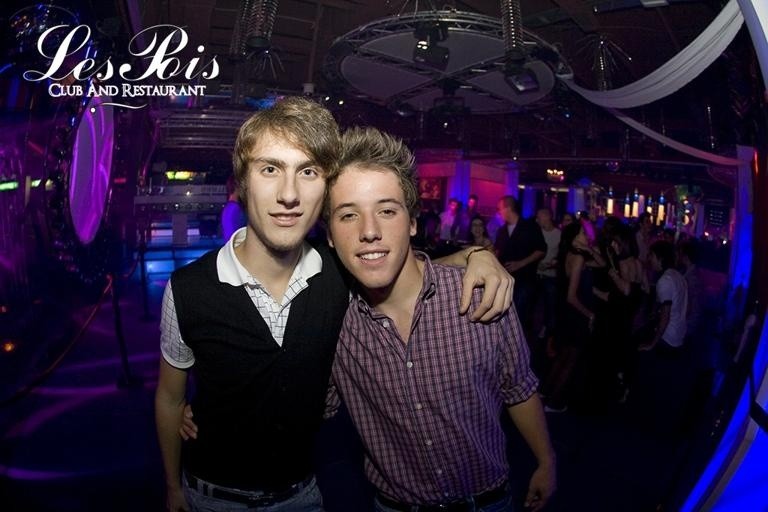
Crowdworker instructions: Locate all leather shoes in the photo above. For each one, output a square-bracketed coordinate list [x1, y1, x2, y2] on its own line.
[537, 392, 569, 414]
[616, 371, 632, 405]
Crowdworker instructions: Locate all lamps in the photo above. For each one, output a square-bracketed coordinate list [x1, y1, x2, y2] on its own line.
[412, 24, 537, 150]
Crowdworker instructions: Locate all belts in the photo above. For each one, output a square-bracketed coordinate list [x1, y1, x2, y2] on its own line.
[183, 468, 317, 508]
[373, 486, 510, 512]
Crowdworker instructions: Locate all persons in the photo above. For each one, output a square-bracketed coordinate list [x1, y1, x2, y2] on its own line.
[418, 181, 720, 419]
[145, 92, 523, 510]
[168, 122, 564, 510]
